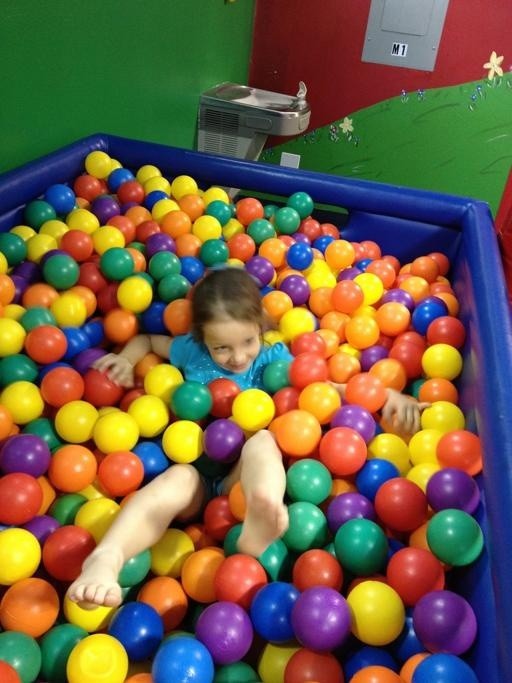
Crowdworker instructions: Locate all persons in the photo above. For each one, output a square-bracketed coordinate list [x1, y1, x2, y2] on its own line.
[64, 267, 430, 611]
